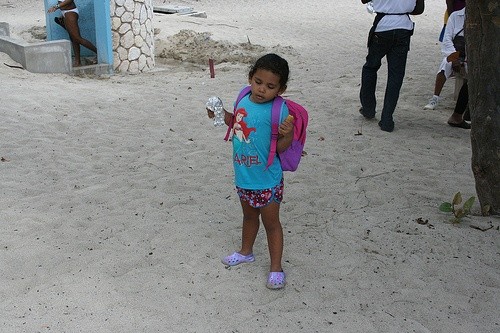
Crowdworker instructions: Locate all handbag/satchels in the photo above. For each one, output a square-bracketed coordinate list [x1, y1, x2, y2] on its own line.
[452, 27, 466, 58]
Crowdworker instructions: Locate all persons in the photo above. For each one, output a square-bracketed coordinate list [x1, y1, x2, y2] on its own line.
[206, 53, 294, 289]
[423, 0, 471, 129]
[360, 0, 425, 132]
[48, 0, 97, 68]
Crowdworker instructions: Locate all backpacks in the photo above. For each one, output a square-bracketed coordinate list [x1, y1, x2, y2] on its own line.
[225, 86, 308, 172]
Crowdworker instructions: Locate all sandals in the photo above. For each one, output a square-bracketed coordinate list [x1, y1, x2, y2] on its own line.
[222, 250, 255, 266]
[266, 268, 286, 288]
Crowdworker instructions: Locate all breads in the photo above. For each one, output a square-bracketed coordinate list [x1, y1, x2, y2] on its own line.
[286, 115, 294, 122]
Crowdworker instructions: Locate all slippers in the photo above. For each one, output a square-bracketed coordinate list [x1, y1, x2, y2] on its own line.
[447, 120, 471, 130]
[463, 114, 472, 121]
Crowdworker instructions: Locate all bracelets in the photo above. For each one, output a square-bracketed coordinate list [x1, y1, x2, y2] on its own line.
[56, 4, 60, 9]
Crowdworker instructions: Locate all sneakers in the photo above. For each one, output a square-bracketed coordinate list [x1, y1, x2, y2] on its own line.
[424, 97, 438, 110]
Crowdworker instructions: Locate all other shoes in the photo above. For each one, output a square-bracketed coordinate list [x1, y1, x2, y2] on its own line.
[359, 107, 375, 119]
[378, 120, 394, 132]
